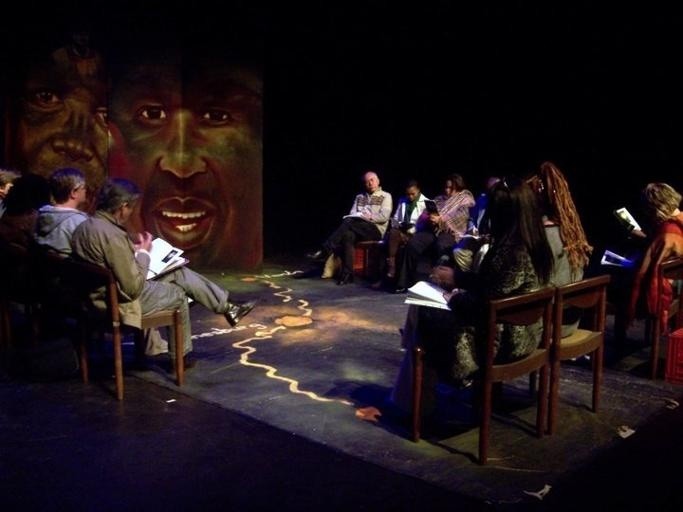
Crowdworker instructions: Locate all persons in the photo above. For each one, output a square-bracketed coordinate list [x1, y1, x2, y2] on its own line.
[307, 172, 392, 285]
[109, 34, 264, 273]
[386, 164, 594, 422]
[595, 182, 683, 365]
[0, 167, 258, 371]
[2, 34, 116, 199]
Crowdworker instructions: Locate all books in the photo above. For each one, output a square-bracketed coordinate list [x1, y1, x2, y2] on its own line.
[136, 238, 189, 281]
[403, 280, 453, 311]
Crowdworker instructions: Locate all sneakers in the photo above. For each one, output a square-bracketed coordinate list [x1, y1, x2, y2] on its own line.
[147, 338, 193, 365]
[312, 248, 410, 293]
[355, 403, 412, 423]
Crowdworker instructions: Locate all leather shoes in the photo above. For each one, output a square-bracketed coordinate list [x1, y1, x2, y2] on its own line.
[224, 296, 258, 327]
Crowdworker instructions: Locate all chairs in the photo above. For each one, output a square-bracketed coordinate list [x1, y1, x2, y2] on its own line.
[0, 238, 184, 402]
